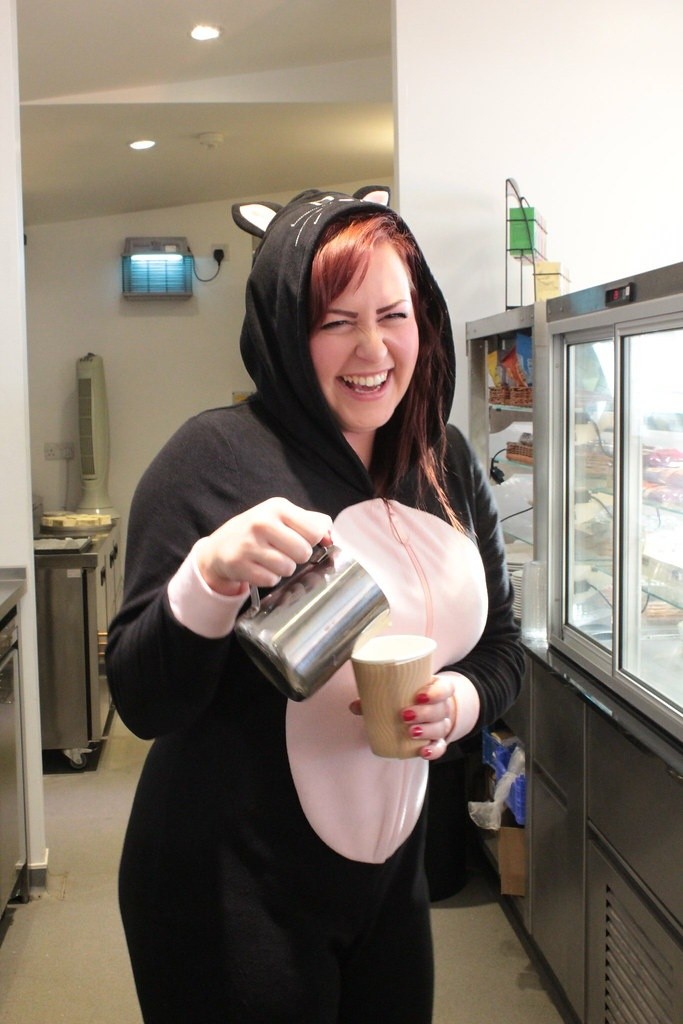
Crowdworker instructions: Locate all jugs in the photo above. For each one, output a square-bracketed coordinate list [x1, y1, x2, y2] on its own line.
[234, 541, 390, 702]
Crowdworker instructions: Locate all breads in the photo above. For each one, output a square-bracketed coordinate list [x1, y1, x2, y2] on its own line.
[42, 513, 111, 528]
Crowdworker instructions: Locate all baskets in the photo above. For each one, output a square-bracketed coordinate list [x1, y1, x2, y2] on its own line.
[510, 386, 533, 407]
[505, 442, 533, 463]
[489, 386, 510, 405]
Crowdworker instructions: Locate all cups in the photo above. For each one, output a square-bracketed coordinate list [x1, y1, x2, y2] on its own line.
[351, 634, 436, 758]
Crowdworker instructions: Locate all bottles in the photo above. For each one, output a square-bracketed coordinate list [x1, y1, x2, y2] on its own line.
[506, 554, 531, 622]
[520, 561, 547, 640]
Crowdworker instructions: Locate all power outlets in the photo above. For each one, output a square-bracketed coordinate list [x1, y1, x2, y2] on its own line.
[212, 243, 230, 263]
[45, 442, 73, 459]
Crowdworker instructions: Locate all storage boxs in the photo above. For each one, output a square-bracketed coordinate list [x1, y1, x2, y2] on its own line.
[482, 729, 515, 768]
[493, 751, 526, 824]
[496, 827, 526, 896]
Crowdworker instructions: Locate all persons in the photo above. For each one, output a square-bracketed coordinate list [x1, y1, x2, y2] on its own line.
[103, 185, 524, 1024]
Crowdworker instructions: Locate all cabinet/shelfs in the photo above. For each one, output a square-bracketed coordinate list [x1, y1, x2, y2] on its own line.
[34, 536, 120, 751]
[487, 402, 682, 612]
